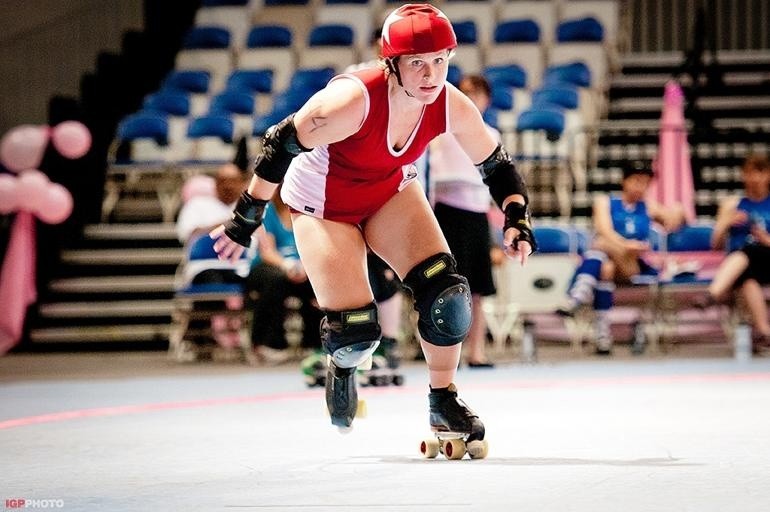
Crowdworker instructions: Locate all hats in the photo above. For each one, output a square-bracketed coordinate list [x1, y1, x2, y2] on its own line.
[622, 160, 653, 176]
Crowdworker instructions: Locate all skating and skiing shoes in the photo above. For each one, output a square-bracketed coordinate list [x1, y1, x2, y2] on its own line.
[326, 360, 358, 426]
[420, 383, 487, 459]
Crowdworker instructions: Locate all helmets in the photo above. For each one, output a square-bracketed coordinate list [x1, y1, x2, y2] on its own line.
[381, 3, 458, 57]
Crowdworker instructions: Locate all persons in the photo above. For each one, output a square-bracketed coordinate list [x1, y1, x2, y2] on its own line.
[554, 160, 654, 355]
[688, 158, 769, 350]
[209, 5, 538, 441]
[174, 76, 501, 384]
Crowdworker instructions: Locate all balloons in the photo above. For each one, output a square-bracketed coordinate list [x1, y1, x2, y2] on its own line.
[0, 124, 50, 174]
[50, 120, 93, 158]
[36, 181, 74, 225]
[0, 174, 16, 215]
[15, 167, 51, 214]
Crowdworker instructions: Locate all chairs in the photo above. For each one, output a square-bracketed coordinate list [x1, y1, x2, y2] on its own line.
[176, 209, 769, 360]
[99, 1, 624, 197]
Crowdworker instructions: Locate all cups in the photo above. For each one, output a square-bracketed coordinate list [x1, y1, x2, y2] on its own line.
[733, 325, 753, 359]
